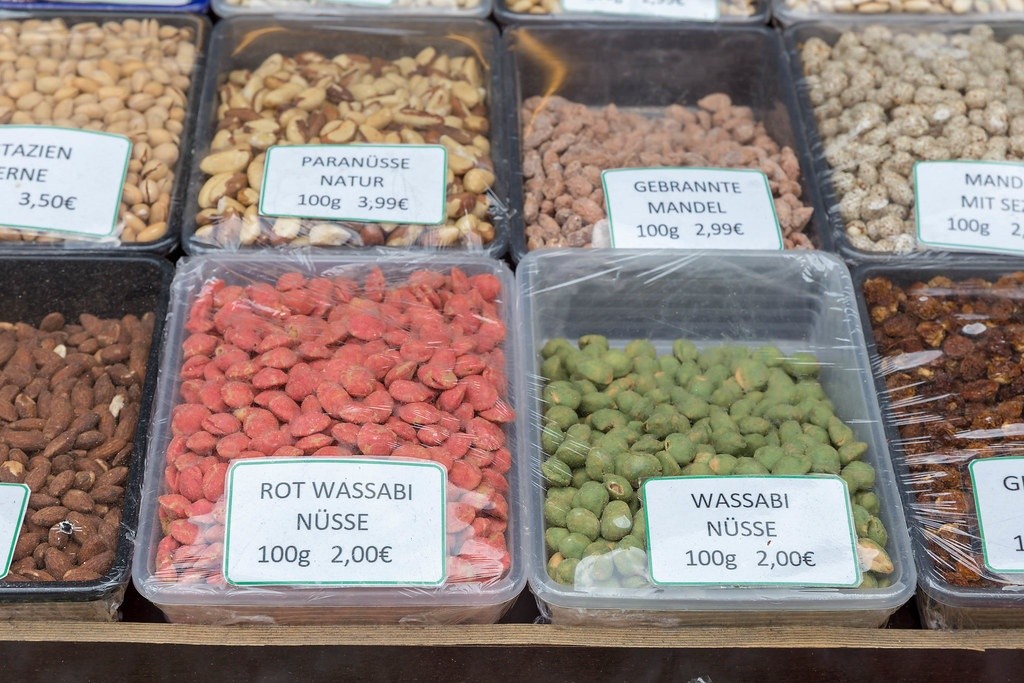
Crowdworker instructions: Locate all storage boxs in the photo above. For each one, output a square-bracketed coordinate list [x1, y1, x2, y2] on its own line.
[0, 1, 1023, 629]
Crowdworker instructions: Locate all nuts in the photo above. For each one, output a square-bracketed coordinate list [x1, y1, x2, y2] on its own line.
[0, 0, 1024, 584]
